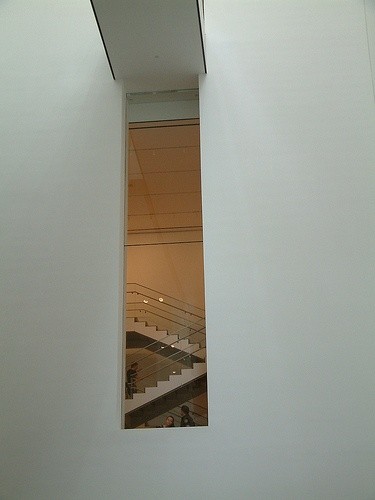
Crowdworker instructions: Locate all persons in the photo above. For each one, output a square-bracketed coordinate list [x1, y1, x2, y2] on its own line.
[180, 405, 195, 426]
[159, 416, 174, 427]
[126, 361, 138, 396]
